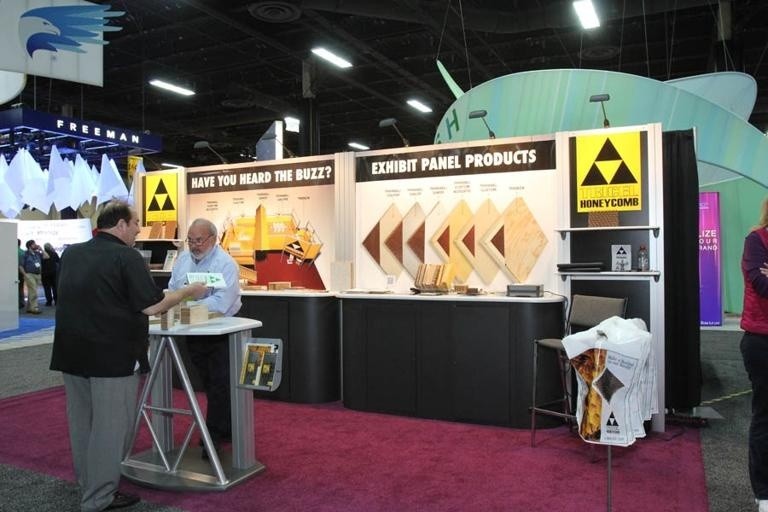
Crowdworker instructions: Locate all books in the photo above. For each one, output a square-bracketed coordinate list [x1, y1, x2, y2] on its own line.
[556, 260, 605, 271]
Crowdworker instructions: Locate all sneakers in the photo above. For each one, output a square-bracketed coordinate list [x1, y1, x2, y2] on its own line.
[20, 303, 57, 312]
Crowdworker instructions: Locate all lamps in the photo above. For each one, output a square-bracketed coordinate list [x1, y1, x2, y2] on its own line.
[193, 140, 229, 164]
[468, 109, 496, 139]
[378, 119, 410, 148]
[589, 93, 612, 129]
[261, 133, 297, 158]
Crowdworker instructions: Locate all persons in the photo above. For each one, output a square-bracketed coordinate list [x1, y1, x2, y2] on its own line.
[19, 240, 51, 314]
[167, 218, 244, 458]
[18, 239, 26, 308]
[735, 197, 768, 511]
[47, 200, 213, 512]
[41, 242, 59, 307]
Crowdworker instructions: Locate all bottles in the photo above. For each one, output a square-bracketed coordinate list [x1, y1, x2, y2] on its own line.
[637, 245, 649, 273]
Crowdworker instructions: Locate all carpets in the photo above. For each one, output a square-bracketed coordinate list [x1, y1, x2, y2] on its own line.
[0, 318, 55, 352]
[1, 382, 709, 512]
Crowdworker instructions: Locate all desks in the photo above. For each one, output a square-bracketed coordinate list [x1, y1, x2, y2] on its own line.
[119, 317, 266, 492]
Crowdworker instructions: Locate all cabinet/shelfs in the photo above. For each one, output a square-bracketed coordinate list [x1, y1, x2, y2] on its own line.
[559, 224, 660, 284]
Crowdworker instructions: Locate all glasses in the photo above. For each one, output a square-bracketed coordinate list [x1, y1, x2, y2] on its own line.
[185, 233, 213, 247]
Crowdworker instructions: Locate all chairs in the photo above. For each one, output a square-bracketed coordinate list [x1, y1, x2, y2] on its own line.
[527, 293, 629, 464]
